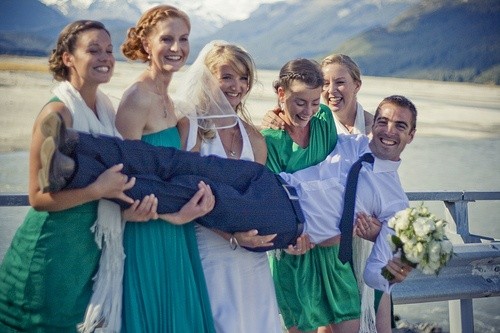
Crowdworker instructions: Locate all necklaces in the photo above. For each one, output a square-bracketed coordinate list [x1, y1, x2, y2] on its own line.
[220, 125, 237, 157]
[156, 88, 169, 119]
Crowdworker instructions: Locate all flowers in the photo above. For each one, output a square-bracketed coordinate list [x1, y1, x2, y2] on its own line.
[380, 203, 456, 281]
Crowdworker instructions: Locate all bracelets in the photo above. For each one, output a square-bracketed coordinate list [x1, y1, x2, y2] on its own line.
[228, 233, 237, 251]
[233, 234, 241, 249]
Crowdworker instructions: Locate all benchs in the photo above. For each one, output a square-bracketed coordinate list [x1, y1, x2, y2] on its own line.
[385, 189, 500, 333]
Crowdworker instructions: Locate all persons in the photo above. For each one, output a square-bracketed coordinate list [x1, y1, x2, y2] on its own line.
[39, 93, 417, 295]
[259, 58, 361, 333]
[261, 52, 381, 332]
[115, 4, 216, 333]
[177, 40, 313, 333]
[0, 17, 159, 333]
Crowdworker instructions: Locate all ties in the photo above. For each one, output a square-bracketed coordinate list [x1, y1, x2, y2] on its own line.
[337, 152, 375, 264]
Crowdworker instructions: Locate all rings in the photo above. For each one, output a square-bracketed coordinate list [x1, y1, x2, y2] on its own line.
[297, 249, 302, 251]
[400, 268, 404, 272]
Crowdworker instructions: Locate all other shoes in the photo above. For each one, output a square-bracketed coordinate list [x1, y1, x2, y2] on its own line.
[37, 136, 75, 193]
[39, 111, 80, 154]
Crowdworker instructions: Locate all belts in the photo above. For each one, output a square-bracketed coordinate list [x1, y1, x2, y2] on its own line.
[275, 174, 305, 246]
